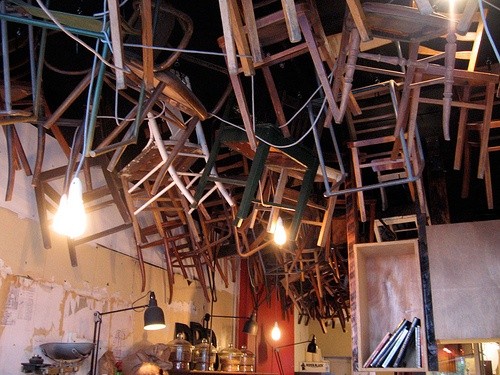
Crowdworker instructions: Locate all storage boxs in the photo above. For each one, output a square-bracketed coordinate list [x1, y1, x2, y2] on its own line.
[353, 240, 428, 372]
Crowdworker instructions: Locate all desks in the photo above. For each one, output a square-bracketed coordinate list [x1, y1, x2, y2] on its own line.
[159, 368, 279, 375]
[300, 360, 330, 372]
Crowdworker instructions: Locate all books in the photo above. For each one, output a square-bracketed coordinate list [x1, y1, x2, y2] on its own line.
[363, 317, 421, 368]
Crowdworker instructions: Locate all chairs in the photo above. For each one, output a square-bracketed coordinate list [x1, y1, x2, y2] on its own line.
[0, 0, 500, 333]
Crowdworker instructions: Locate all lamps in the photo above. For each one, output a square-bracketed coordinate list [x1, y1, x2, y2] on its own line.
[49, 125, 88, 240]
[91, 290, 166, 375]
[442, 345, 452, 354]
[270, 177, 287, 245]
[204, 310, 258, 335]
[273, 334, 319, 375]
[271, 293, 282, 340]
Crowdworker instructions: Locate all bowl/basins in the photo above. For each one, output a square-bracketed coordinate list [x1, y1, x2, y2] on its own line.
[40, 343, 97, 359]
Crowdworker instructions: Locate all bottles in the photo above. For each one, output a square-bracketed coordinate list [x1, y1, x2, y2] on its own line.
[169, 331, 193, 370]
[194, 340, 217, 371]
[240, 347, 255, 372]
[220, 343, 241, 372]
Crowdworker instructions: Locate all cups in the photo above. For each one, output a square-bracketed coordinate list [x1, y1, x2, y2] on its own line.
[456, 358, 469, 374]
